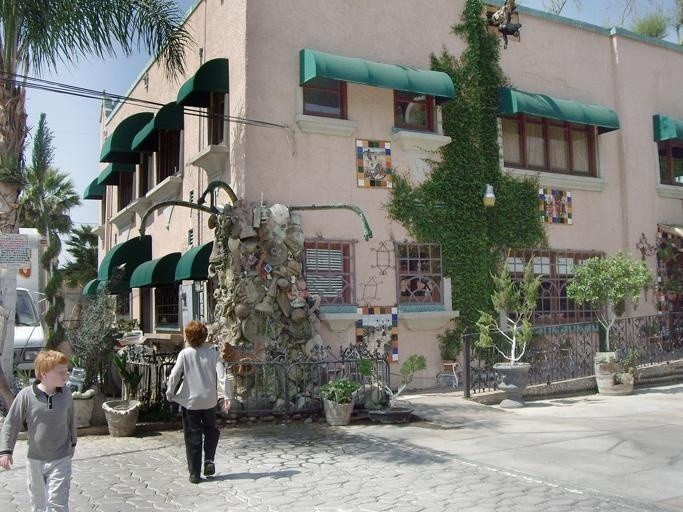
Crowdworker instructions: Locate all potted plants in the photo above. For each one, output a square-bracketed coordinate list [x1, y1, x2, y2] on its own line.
[436, 322, 465, 372]
[317, 352, 427, 425]
[99, 350, 142, 435]
[471, 245, 545, 409]
[563, 250, 653, 395]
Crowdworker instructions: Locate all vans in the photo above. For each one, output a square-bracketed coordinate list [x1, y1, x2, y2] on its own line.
[13, 287, 45, 377]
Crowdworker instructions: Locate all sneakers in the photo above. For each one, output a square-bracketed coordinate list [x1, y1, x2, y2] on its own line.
[204, 462, 215, 476]
[189, 474, 200, 483]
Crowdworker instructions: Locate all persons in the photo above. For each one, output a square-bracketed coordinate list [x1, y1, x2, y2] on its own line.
[166, 319, 232, 483]
[0, 349, 78, 512]
